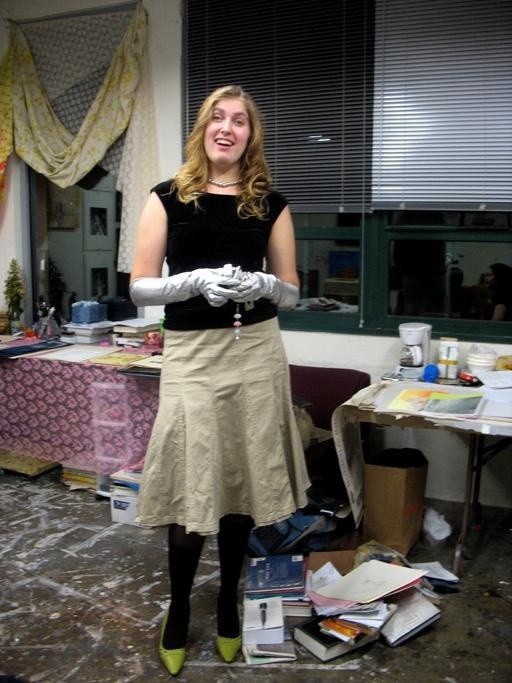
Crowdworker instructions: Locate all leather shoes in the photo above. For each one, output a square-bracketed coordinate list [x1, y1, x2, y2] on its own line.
[158, 608, 187, 676]
[215, 634, 242, 663]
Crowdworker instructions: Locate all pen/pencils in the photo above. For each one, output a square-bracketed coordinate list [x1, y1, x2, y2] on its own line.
[34, 295, 56, 340]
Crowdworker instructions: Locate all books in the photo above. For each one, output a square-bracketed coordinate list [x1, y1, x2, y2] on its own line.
[371, 365, 512, 425]
[1, 316, 164, 359]
[238, 543, 443, 666]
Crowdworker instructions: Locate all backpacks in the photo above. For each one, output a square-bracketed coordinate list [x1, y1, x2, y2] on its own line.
[247, 468, 355, 557]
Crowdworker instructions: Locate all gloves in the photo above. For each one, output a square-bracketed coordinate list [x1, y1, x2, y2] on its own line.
[229, 270, 299, 311]
[129, 266, 241, 307]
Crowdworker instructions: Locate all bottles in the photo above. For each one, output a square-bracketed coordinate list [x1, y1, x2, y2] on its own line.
[436, 336, 459, 380]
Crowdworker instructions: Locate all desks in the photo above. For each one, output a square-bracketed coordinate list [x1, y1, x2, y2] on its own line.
[0, 338, 162, 475]
[344, 372, 512, 578]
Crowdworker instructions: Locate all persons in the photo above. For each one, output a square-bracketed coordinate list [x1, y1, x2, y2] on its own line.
[389, 208, 449, 317]
[93, 214, 107, 236]
[485, 262, 512, 320]
[129, 82, 314, 677]
[448, 266, 487, 320]
[95, 271, 111, 300]
[324, 159, 363, 245]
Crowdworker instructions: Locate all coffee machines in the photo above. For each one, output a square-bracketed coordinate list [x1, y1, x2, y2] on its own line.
[395, 323, 432, 378]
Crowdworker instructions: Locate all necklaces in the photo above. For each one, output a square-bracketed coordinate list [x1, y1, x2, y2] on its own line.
[205, 172, 246, 188]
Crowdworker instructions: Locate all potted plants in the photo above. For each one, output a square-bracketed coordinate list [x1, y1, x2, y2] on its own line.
[3, 259, 26, 333]
